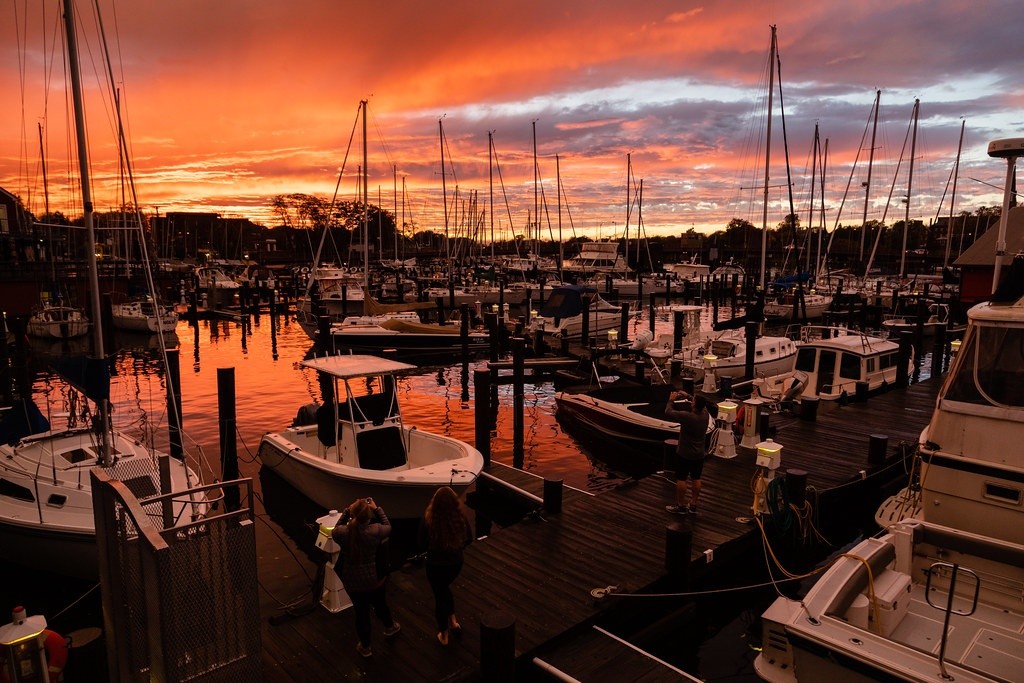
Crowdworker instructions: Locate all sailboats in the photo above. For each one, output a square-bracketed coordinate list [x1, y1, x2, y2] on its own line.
[24, 23, 970, 445]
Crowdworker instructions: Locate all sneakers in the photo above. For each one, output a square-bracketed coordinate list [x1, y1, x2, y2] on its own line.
[382, 622, 402, 636]
[355, 642, 373, 656]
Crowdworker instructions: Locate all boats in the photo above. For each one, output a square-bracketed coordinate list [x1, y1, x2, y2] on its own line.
[0, 0, 213, 547]
[256, 350, 484, 522]
[748, 130, 1024, 683]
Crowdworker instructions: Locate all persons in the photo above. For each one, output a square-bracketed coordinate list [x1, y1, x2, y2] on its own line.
[665, 390, 709, 515]
[425, 487, 474, 645]
[332, 496, 402, 657]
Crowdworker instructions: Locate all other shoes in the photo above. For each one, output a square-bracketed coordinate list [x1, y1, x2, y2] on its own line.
[435, 630, 450, 645]
[687, 504, 698, 516]
[665, 504, 689, 516]
[448, 621, 463, 631]
